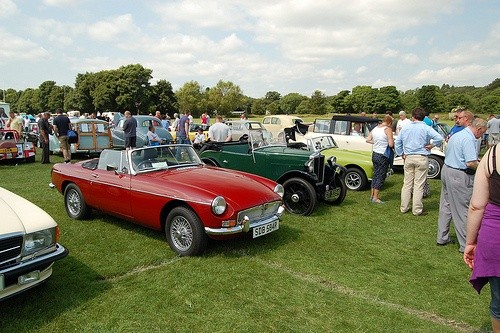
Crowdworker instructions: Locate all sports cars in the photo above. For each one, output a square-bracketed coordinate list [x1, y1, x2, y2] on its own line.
[48, 144, 286, 258]
[180, 127, 348, 218]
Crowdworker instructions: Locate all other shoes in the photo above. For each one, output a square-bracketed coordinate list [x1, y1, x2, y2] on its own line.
[370, 197, 385, 203]
[437, 242, 447, 246]
[65, 160, 71, 164]
[423, 193, 428, 198]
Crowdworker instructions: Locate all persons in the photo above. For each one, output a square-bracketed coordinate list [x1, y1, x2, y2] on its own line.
[53, 108, 72, 165]
[85, 112, 115, 132]
[146, 111, 249, 152]
[6, 110, 43, 142]
[121, 111, 137, 148]
[38, 112, 52, 163]
[366, 115, 395, 204]
[355, 105, 500, 333]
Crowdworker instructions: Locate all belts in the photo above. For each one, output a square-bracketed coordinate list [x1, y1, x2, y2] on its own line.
[445, 164, 466, 172]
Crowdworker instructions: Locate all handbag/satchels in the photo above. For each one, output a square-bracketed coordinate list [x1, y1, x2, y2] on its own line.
[67, 130, 78, 144]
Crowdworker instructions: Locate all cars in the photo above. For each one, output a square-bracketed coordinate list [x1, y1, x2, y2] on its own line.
[0, 88, 453, 193]
[0, 187, 70, 302]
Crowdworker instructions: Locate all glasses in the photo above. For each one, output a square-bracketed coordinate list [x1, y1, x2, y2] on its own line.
[454, 115, 458, 118]
[458, 116, 468, 120]
